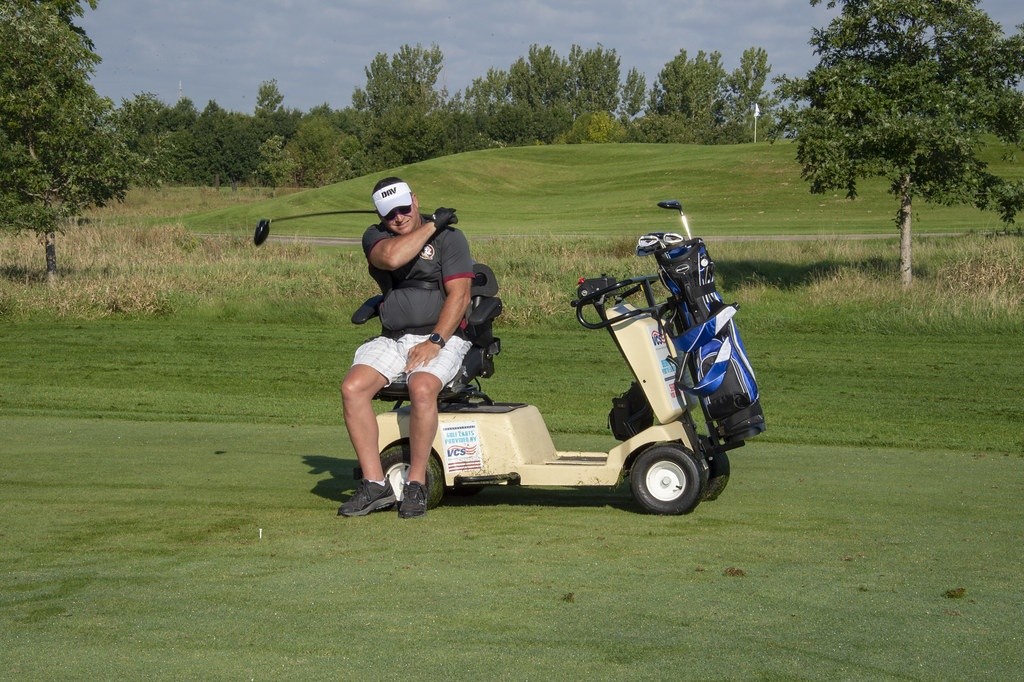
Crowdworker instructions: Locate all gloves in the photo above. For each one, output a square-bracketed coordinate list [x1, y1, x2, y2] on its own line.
[426, 206, 456, 231]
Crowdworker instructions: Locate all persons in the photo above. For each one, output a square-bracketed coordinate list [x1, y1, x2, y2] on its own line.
[337, 176, 475, 518]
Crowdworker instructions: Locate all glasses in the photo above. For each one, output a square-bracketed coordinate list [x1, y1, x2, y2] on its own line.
[384, 205, 412, 221]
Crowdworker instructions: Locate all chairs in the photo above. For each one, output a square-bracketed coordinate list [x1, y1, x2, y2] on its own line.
[350, 263, 502, 400]
[354, 263, 503, 401]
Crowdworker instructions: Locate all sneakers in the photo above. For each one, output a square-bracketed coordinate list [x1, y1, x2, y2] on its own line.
[398, 481, 428, 518]
[338, 474, 396, 516]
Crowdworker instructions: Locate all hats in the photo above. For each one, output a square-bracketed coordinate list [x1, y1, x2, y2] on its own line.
[372, 182, 412, 217]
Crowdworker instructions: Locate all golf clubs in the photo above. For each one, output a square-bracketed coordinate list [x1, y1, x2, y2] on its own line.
[637, 200, 693, 259]
[253, 209, 458, 246]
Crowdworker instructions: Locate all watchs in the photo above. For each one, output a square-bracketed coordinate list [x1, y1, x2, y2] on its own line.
[429, 333, 445, 348]
